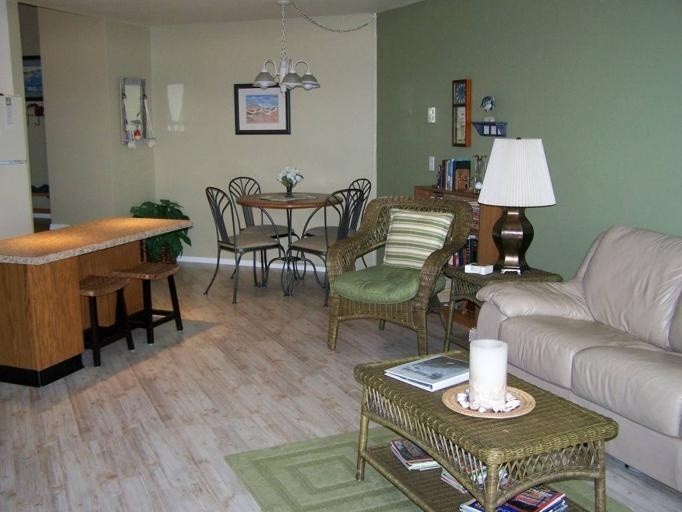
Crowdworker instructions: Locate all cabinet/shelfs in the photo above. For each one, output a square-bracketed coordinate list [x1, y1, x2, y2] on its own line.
[412, 184, 507, 328]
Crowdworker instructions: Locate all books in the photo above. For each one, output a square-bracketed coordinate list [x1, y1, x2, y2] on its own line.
[436, 159, 480, 315]
[390, 438, 568, 512]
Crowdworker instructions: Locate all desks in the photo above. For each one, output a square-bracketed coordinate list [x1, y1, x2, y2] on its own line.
[0, 210, 197, 390]
[442, 262, 564, 354]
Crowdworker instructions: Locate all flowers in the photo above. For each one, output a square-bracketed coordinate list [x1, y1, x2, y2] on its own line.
[274, 164, 305, 189]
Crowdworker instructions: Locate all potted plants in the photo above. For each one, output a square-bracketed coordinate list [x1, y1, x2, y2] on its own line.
[128, 197, 195, 267]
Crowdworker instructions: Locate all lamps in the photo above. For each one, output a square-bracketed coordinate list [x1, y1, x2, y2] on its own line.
[474, 136, 562, 277]
[247, 1, 322, 96]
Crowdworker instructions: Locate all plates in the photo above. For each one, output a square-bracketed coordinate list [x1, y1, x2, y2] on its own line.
[442, 382, 536, 420]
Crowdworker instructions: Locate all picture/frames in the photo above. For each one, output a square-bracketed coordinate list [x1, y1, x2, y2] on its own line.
[21, 54, 45, 103]
[232, 82, 293, 138]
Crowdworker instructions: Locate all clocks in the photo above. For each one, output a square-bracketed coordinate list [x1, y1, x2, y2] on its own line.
[450, 78, 472, 148]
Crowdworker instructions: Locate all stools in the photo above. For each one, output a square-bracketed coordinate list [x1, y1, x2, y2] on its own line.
[78, 271, 142, 370]
[110, 256, 186, 349]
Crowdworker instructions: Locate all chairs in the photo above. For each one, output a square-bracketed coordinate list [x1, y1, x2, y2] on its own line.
[202, 177, 372, 310]
[324, 195, 475, 358]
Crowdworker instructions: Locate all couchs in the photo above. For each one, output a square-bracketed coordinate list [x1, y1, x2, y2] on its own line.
[475, 222, 681, 499]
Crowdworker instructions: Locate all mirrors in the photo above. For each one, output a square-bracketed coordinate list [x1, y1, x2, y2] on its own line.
[118, 73, 156, 149]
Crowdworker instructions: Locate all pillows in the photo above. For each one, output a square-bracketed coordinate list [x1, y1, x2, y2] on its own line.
[381, 206, 456, 272]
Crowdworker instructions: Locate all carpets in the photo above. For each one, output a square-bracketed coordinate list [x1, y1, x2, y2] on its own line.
[222, 424, 640, 511]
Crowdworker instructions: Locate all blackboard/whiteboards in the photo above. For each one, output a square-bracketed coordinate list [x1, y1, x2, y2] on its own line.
[0, 94, 27, 164]
